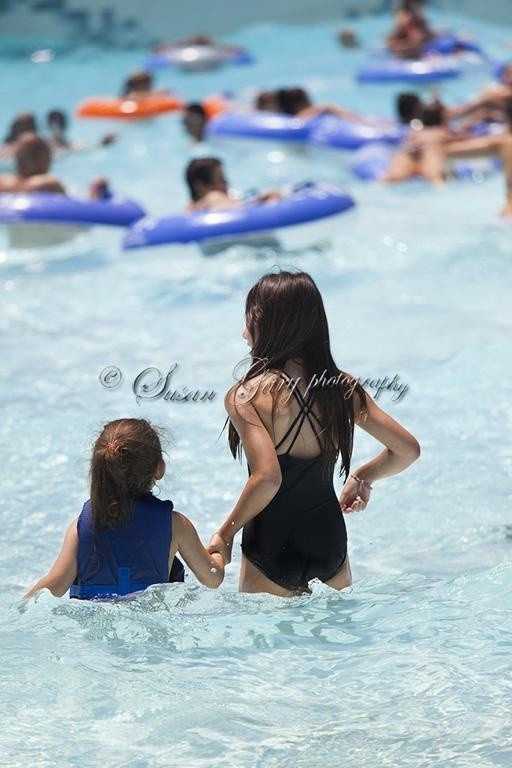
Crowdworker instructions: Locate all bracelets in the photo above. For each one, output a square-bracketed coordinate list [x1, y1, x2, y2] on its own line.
[350, 473, 372, 494]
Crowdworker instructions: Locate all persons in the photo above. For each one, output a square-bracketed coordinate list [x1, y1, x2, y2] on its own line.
[152, 34, 236, 52]
[182, 104, 205, 142]
[383, 8, 428, 61]
[1, 131, 111, 200]
[23, 415, 226, 600]
[184, 156, 283, 217]
[201, 267, 422, 596]
[383, 62, 512, 221]
[256, 87, 367, 128]
[43, 111, 72, 156]
[122, 70, 154, 98]
[340, 31, 360, 49]
[1, 114, 40, 159]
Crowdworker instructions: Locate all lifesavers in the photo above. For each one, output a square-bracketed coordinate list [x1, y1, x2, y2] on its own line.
[147, 46, 254, 69]
[0, 190, 143, 225]
[355, 37, 483, 81]
[78, 92, 325, 140]
[122, 176, 356, 249]
[310, 113, 509, 183]
